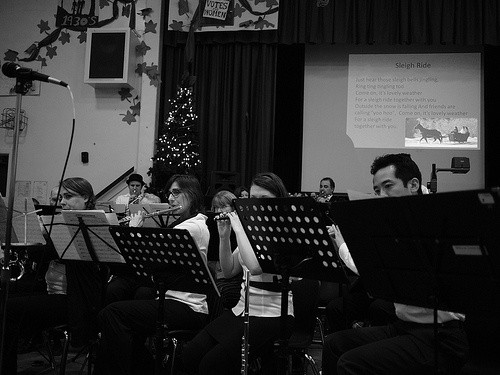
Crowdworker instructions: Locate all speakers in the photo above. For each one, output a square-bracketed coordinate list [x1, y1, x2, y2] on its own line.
[83, 28, 139, 89]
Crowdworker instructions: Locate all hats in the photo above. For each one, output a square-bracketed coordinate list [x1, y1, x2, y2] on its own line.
[126, 174, 145, 186]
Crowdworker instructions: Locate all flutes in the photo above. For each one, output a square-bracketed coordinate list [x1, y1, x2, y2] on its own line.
[117, 206, 184, 225]
[215, 215, 229, 221]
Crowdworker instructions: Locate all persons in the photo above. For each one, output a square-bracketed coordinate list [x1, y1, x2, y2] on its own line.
[293, 177, 346, 217]
[319, 153, 472, 375]
[169, 171, 305, 374]
[0, 172, 252, 375]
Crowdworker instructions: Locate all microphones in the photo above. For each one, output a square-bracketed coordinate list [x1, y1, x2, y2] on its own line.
[2, 61, 68, 88]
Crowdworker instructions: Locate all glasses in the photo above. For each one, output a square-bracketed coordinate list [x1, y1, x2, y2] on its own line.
[164, 189, 187, 198]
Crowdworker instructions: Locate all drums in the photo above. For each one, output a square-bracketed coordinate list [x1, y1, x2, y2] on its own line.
[2, 242, 45, 282]
